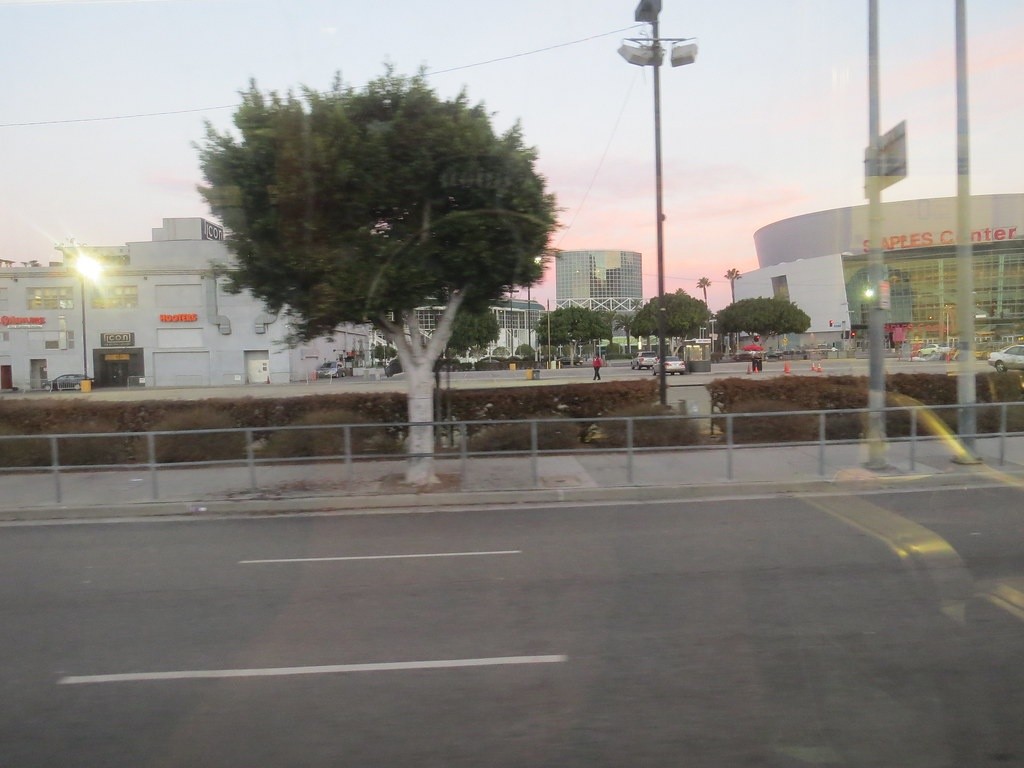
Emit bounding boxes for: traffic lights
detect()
[755,336,759,343]
[851,332,855,339]
[830,319,833,327]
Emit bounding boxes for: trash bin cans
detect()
[80,379,92,393]
[532,370,540,380]
[752,359,762,371]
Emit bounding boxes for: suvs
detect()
[631,351,658,370]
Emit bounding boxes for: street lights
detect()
[527,255,543,347]
[77,259,91,379]
[698,328,707,339]
[709,319,717,353]
[618,0,698,405]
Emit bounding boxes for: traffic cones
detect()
[784,361,790,373]
[747,364,753,374]
[754,365,758,373]
[817,362,822,372]
[811,362,816,371]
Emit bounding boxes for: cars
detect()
[41,374,95,391]
[988,344,1024,373]
[917,343,951,357]
[651,356,686,375]
[555,357,577,365]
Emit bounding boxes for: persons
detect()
[592,353,602,380]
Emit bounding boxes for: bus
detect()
[949,342,994,360]
